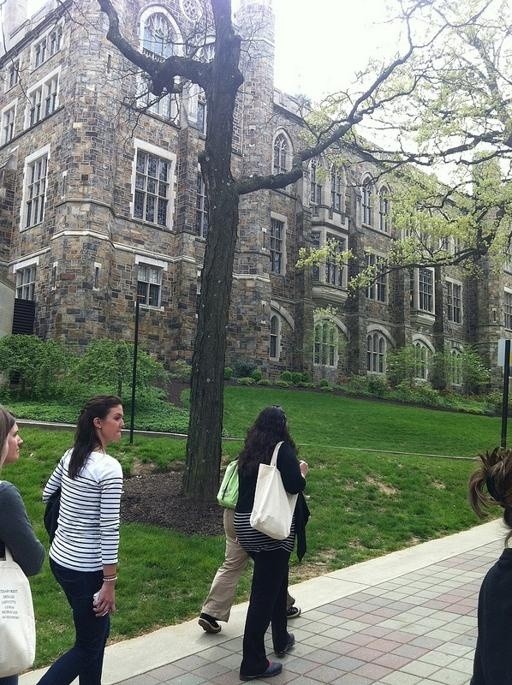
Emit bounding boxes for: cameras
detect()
[93,590,109,617]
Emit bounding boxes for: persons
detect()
[1,405,11,472]
[1,404,49,684]
[196,450,302,634]
[463,447,511,685]
[232,403,309,681]
[35,394,127,685]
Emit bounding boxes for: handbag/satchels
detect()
[0,560,36,678]
[43,486,64,542]
[217,459,241,508]
[250,463,301,542]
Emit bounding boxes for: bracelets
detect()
[100,573,119,584]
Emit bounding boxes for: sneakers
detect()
[286,606,302,619]
[198,612,222,635]
[239,662,283,682]
[276,631,295,659]
